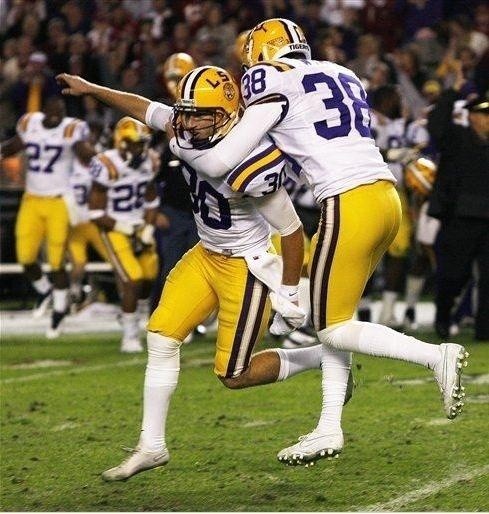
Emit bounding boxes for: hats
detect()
[463,94,488,115]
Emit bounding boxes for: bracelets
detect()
[168,136,186,157]
[280,285,301,305]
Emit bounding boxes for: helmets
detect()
[114,114,151,162]
[161,52,197,81]
[172,64,240,150]
[230,16,312,71]
[402,153,439,197]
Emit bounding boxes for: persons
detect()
[52,66,356,486]
[163,16,469,466]
[1,2,489,349]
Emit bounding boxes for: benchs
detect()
[1,259,118,307]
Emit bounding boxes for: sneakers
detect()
[117,309,145,357]
[33,287,53,314]
[274,428,346,466]
[46,301,70,343]
[433,341,470,420]
[103,444,173,483]
